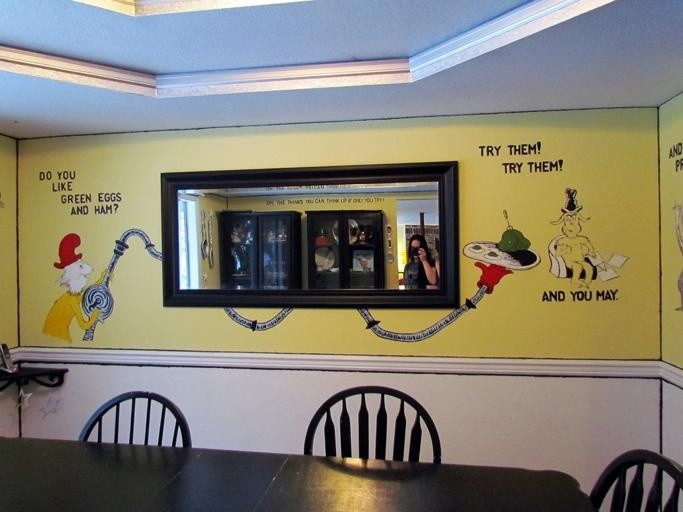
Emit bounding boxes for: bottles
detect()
[359,231,366,245]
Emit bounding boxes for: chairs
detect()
[305,385,441,465]
[77,390,191,450]
[590,450,683,511]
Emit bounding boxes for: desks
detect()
[0,436,589,511]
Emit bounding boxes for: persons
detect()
[404,234,438,290]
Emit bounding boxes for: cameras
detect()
[409,247,419,256]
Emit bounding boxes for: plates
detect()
[314,247,335,270]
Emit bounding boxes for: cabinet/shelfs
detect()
[306,210,384,290]
[220,210,301,290]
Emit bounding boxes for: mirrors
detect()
[161,162,460,309]
